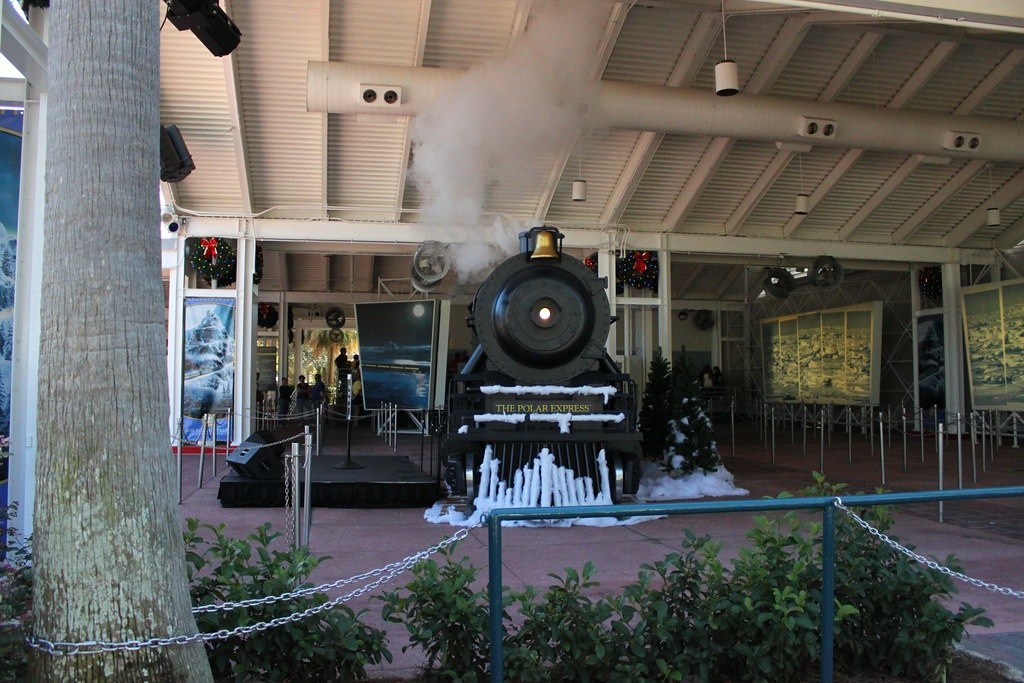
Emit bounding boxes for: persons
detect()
[312,374,325,425]
[699,364,723,387]
[279,378,291,425]
[449,350,470,369]
[297,375,316,427]
[256,383,264,417]
[335,348,348,400]
[351,355,361,380]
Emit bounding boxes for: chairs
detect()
[256,390,276,430]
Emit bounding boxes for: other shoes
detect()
[309,423,317,427]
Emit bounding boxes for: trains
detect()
[442,223,647,511]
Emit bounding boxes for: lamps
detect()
[794,153,810,216]
[159,0,243,59]
[942,130,983,155]
[984,163,1004,228]
[359,82,403,109]
[573,134,586,203]
[798,114,839,141]
[713,0,740,98]
[679,308,695,321]
[160,121,196,184]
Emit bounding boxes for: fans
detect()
[696,310,714,331]
[414,238,453,281]
[810,255,846,293]
[330,329,344,342]
[409,260,442,294]
[761,269,797,302]
[326,307,346,329]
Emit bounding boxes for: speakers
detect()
[225,430,287,480]
